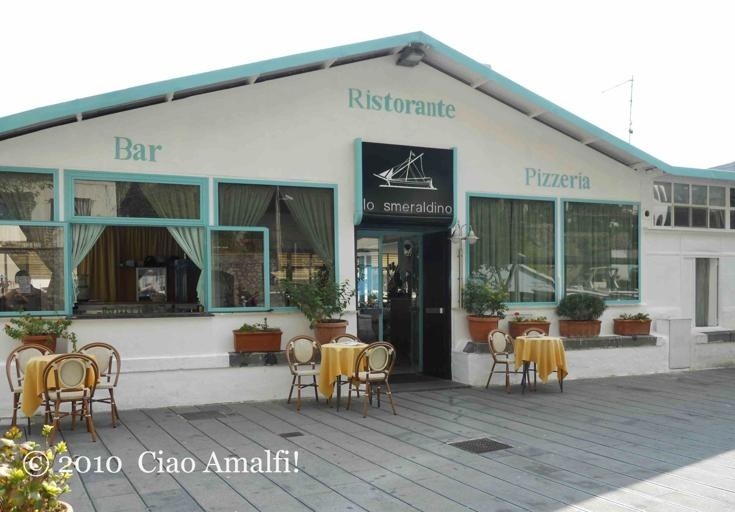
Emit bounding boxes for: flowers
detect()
[457,281,507,343]
[0,424,75,511]
[512,310,547,322]
[1,308,78,354]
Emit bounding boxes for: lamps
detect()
[450,221,480,310]
[396,46,425,67]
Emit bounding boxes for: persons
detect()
[4,271,48,312]
[139,268,160,300]
[610,270,620,291]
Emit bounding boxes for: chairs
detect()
[284,335,334,412]
[76,342,122,430]
[485,329,533,393]
[7,344,57,430]
[331,335,364,398]
[43,353,100,443]
[522,329,547,389]
[348,341,400,418]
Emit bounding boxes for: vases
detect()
[51,501,73,512]
[22,334,56,353]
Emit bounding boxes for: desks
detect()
[20,353,98,431]
[318,342,369,411]
[515,336,569,394]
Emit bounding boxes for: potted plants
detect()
[231,317,282,353]
[557,292,605,337]
[508,320,550,339]
[279,270,355,343]
[613,312,652,336]
